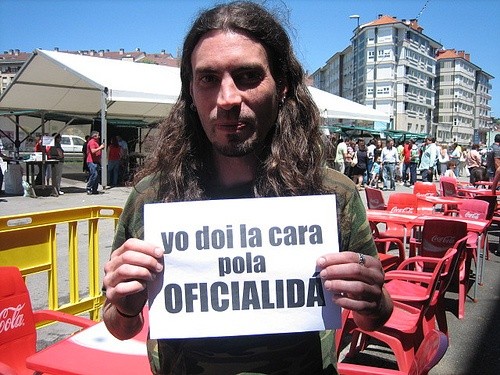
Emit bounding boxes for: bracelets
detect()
[115,306,142,317]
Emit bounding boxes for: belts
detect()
[384,162,393,164]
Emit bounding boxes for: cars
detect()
[49,135,87,152]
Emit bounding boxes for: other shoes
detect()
[380,186,387,191]
[389,187,395,191]
[58,190,64,194]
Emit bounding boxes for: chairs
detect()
[0,262,96,375]
[334,177,500,375]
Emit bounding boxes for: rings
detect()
[359,252,366,265]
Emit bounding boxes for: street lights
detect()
[348,14,360,28]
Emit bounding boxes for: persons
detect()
[101,0,394,375]
[324,131,500,190]
[34,131,131,195]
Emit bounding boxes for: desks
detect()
[367,207,491,233]
[26,306,153,375]
[22,159,60,188]
[419,195,465,207]
[458,187,500,197]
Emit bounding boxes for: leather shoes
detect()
[87,190,92,195]
[92,190,100,194]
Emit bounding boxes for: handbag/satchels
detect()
[350,150,358,167]
